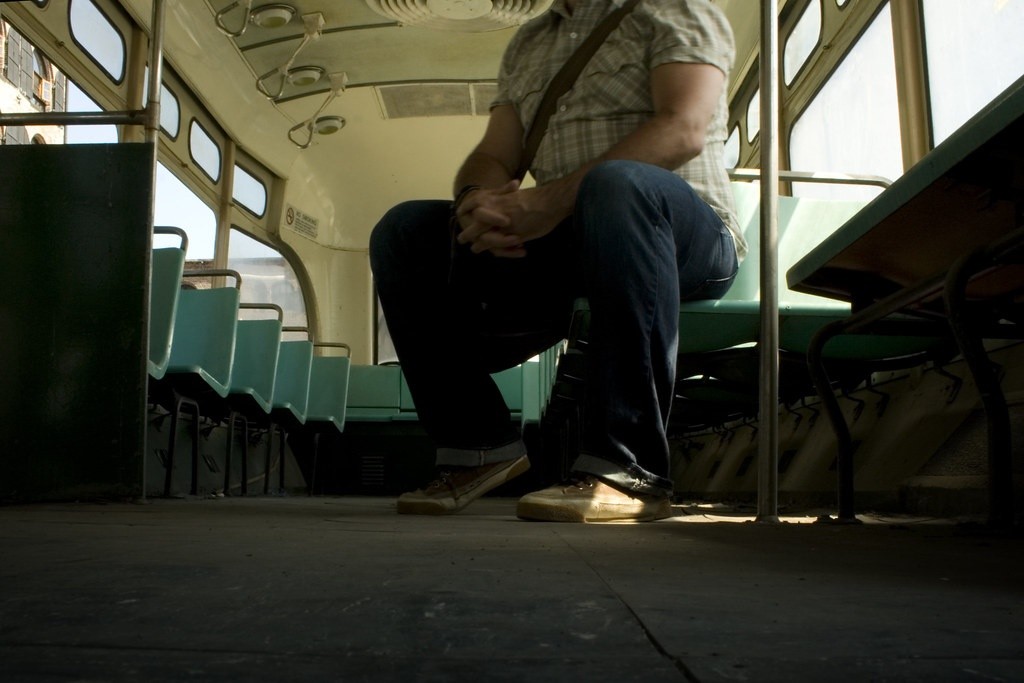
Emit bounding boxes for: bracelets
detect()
[449,184,481,223]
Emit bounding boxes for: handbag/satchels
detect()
[443,214,586,374]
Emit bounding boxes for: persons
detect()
[368,0,748,524]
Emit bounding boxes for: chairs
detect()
[396,371,420,423]
[149,272,242,497]
[786,77,1024,541]
[346,363,402,422]
[290,343,353,496]
[493,364,523,421]
[198,304,283,497]
[239,326,317,497]
[146,228,187,377]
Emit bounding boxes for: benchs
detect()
[518,164,973,524]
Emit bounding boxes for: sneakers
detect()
[517,473,673,523]
[397,453,531,515]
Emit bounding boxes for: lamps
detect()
[307,116,346,136]
[282,66,325,87]
[246,3,297,30]
